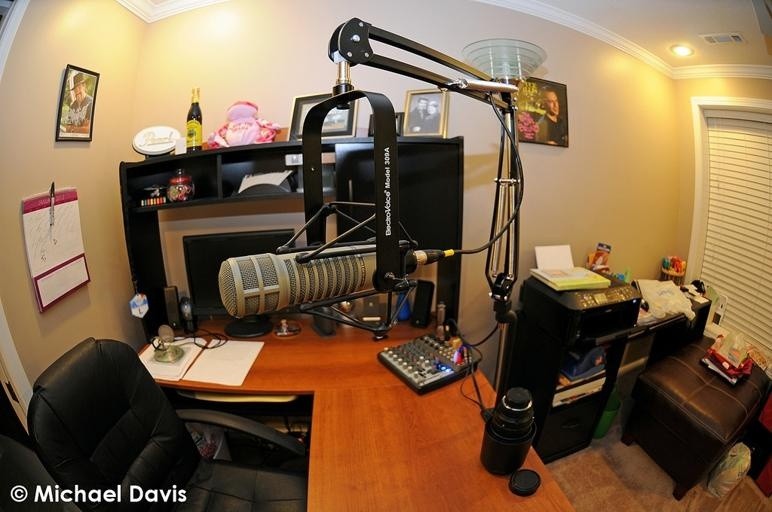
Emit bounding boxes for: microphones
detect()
[216,247,443,321]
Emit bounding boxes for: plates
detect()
[132,125,181,156]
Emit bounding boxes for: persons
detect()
[412,100,442,134]
[532,85,566,146]
[407,97,430,133]
[59,71,93,134]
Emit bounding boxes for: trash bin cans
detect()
[186,423,231,460]
[593,394,622,438]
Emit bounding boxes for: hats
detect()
[66,72,91,91]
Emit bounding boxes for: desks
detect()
[139,316,576,512]
[611,283,712,423]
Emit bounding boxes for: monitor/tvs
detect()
[183,228,295,338]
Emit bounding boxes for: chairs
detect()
[26,336,308,512]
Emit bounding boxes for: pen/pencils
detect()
[50,182,54,226]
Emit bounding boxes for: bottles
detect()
[394,290,411,324]
[479,386,540,477]
[186,87,203,153]
[166,166,198,203]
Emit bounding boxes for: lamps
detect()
[460,38,547,392]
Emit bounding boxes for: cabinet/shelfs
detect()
[119,135,464,341]
[496,265,642,464]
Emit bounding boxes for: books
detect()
[527,266,612,293]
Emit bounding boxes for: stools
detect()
[619,335,772,501]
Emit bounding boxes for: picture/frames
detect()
[367,112,404,137]
[55,64,100,141]
[401,88,449,139]
[285,89,360,141]
[512,75,569,147]
